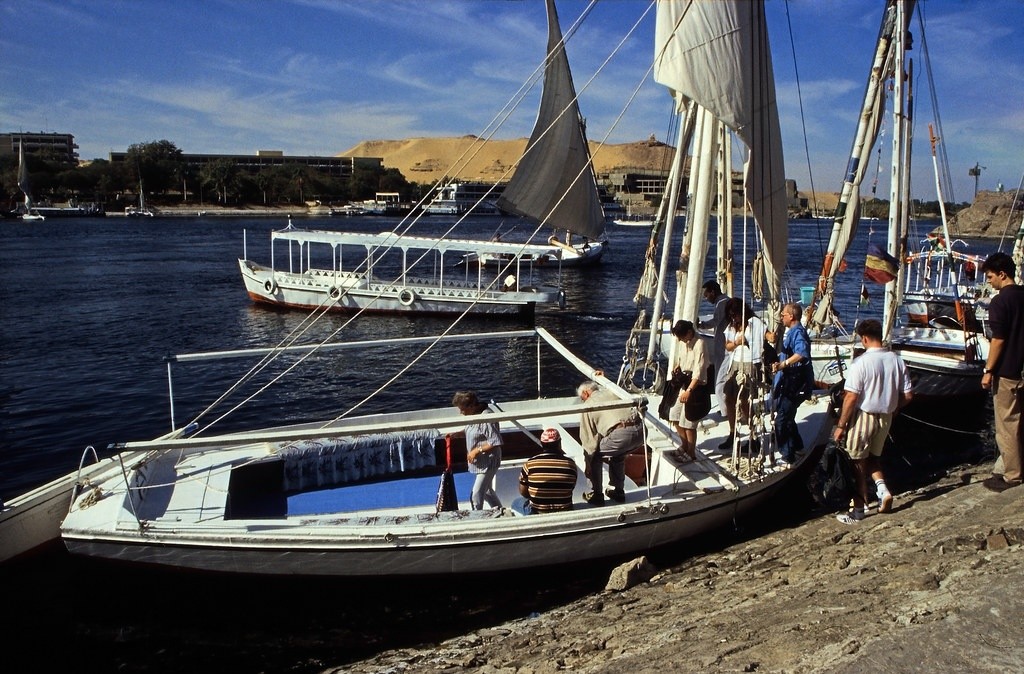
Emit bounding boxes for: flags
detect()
[863,244,900,285]
[859,284,869,306]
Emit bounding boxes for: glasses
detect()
[728,313,740,319]
[781,312,789,317]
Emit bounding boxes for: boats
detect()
[236,213,562,314]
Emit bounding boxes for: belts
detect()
[617,418,642,427]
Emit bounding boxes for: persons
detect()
[452,391,504,510]
[696,279,731,385]
[511,428,578,515]
[834,319,913,525]
[576,371,648,504]
[716,297,778,453]
[669,320,711,463]
[772,303,814,463]
[981,252,1024,490]
[501,271,517,292]
[492,234,501,242]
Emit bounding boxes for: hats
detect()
[541,429,560,442]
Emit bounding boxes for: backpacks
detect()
[817,438,860,500]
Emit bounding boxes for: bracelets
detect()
[837,425,846,429]
[687,387,694,393]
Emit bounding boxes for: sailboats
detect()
[127,163,161,217]
[476,0,610,268]
[56,1,1023,577]
[19,128,46,221]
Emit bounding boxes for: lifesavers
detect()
[583,245,591,256]
[398,288,415,306]
[327,284,343,302]
[829,379,845,416]
[262,277,276,295]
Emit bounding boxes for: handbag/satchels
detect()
[707,363,715,394]
[436,437,458,512]
[782,328,813,393]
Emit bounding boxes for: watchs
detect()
[478,447,483,453]
[983,368,992,374]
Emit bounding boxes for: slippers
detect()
[670,447,697,462]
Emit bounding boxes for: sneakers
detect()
[877,489,893,514]
[836,513,861,525]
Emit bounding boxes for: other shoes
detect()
[605,488,625,503]
[718,436,740,449]
[983,474,1020,492]
[775,458,788,466]
[738,438,760,453]
[795,449,806,460]
[583,492,604,507]
